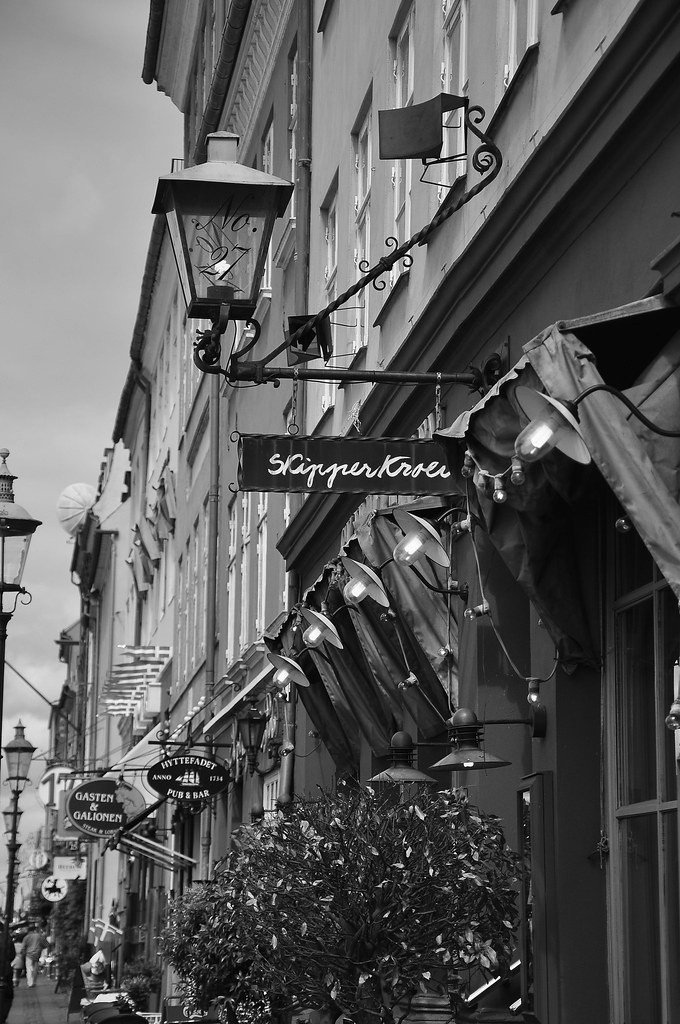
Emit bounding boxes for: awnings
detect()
[100,795,197,874]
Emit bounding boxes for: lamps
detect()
[240,795,323,828]
[237,691,281,776]
[339,551,470,607]
[299,604,360,649]
[139,825,176,843]
[365,732,458,784]
[428,706,546,771]
[267,647,335,688]
[151,130,510,397]
[71,840,97,868]
[512,382,680,462]
[392,506,487,568]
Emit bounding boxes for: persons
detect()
[11,935,26,986]
[20,927,44,988]
[0,921,17,1024]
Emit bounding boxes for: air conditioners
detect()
[132,682,162,735]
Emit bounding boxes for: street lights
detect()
[2,718,38,933]
[0,448,43,774]
